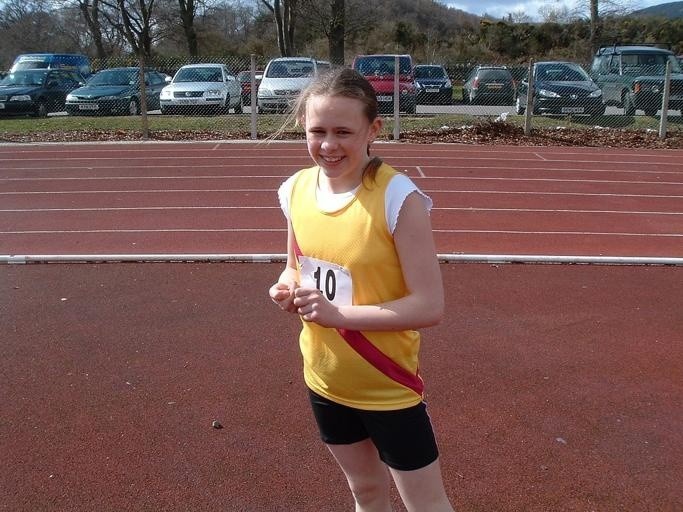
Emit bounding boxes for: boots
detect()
[589,43,683,117]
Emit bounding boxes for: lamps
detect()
[271,65,289,75]
[301,67,310,73]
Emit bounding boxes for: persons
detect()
[266,65,461,512]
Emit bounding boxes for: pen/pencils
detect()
[461,65,517,104]
[351,54,417,115]
[412,65,453,102]
[5,53,93,82]
[256,57,332,113]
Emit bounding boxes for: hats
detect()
[160,64,244,115]
[515,60,607,120]
[237,71,262,94]
[65,67,171,113]
[0,69,89,118]
